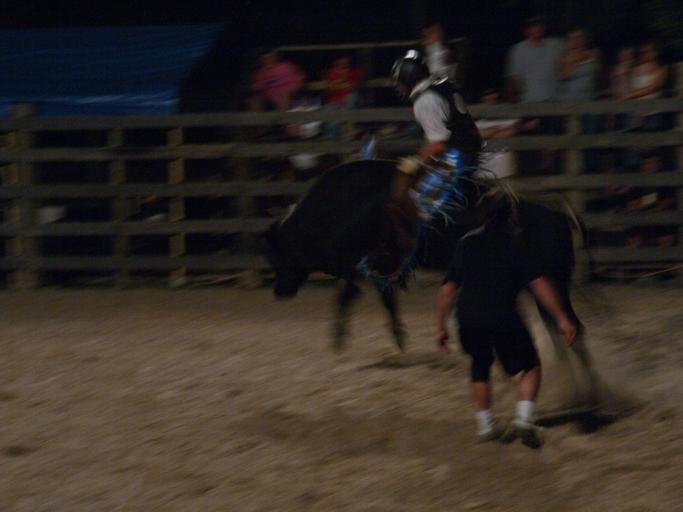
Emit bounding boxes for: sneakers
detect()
[500,422,542,449]
[475,424,499,440]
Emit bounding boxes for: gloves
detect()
[396,153,423,177]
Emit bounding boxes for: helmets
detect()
[391,58,431,90]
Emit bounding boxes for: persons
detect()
[436,188,577,448]
[257,49,427,215]
[473,23,679,248]
[362,27,502,290]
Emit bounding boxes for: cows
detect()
[264,159,603,388]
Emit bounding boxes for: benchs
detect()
[272,0,463,104]
